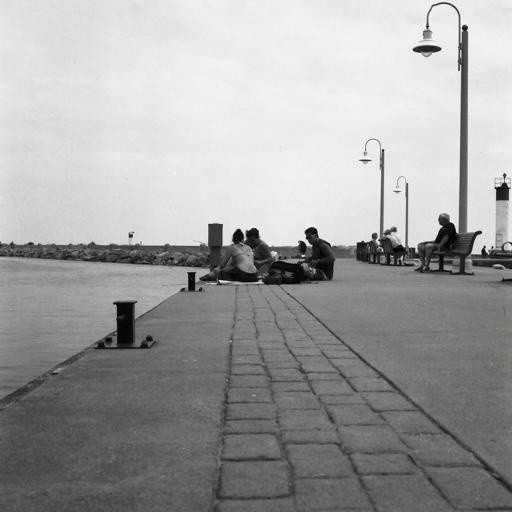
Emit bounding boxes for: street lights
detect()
[360,139,383,253]
[414,3,473,257]
[394,176,414,247]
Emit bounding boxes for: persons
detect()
[370,227,407,265]
[282,227,335,283]
[294,241,307,259]
[243,228,273,277]
[414,213,456,273]
[482,246,487,256]
[199,229,258,282]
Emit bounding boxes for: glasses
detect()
[306,237,311,239]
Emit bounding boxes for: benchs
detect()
[378,238,401,265]
[433,231,483,275]
[368,242,382,263]
